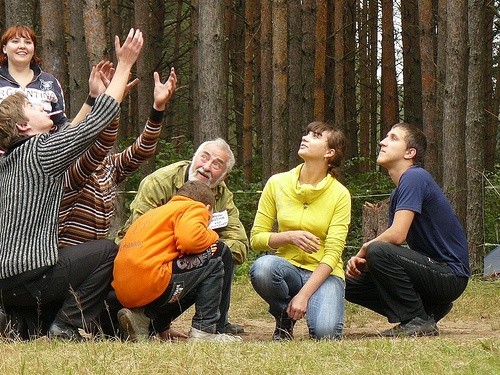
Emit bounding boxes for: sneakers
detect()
[435,303,453,323]
[218,322,243,333]
[383,314,439,337]
[118,306,151,343]
[271,316,297,342]
[190,326,243,344]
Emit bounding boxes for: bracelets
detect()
[85,96,96,106]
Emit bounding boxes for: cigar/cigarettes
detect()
[46,110,62,116]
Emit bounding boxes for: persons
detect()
[250,121,351,342]
[0,25,249,345]
[344,123,471,336]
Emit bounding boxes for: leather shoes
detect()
[50,324,90,342]
